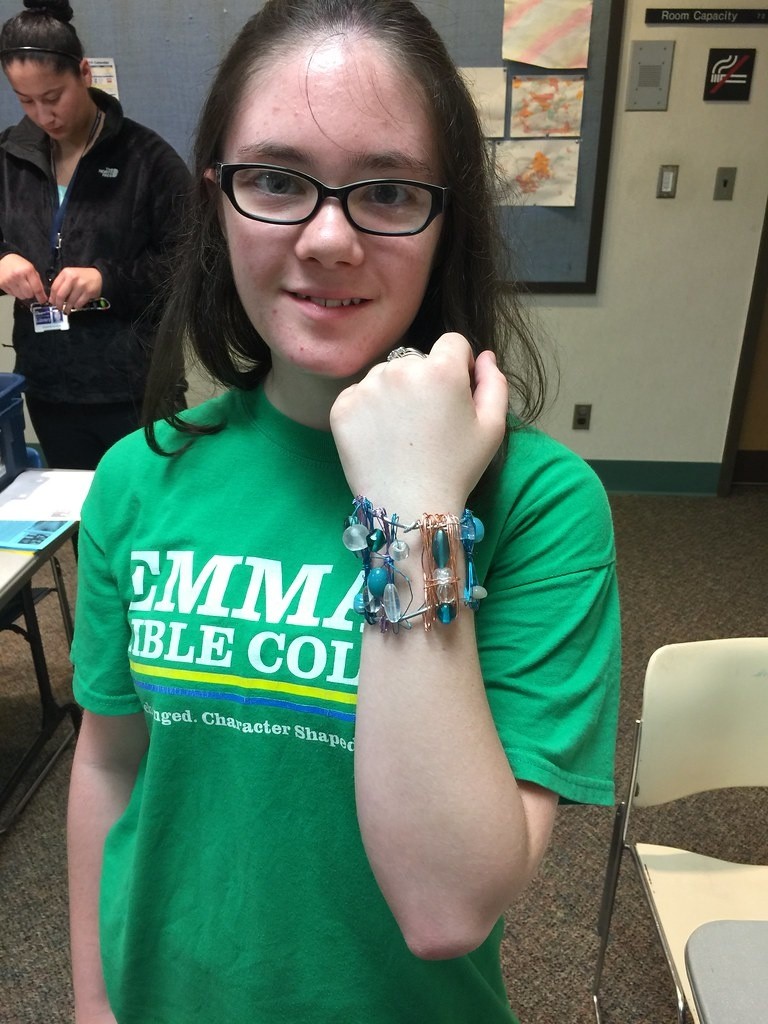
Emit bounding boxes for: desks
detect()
[0,468,98,844]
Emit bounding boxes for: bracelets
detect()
[342,495,489,635]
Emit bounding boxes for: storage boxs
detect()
[0,371,30,494]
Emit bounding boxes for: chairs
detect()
[591,637,768,1024]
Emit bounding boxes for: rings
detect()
[386,347,427,362]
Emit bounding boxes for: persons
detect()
[70,0,624,1024]
[1,0,198,566]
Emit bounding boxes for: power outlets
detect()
[573,404,591,429]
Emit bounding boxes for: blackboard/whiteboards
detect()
[0,1,629,295]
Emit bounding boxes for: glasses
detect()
[210,159,452,239]
